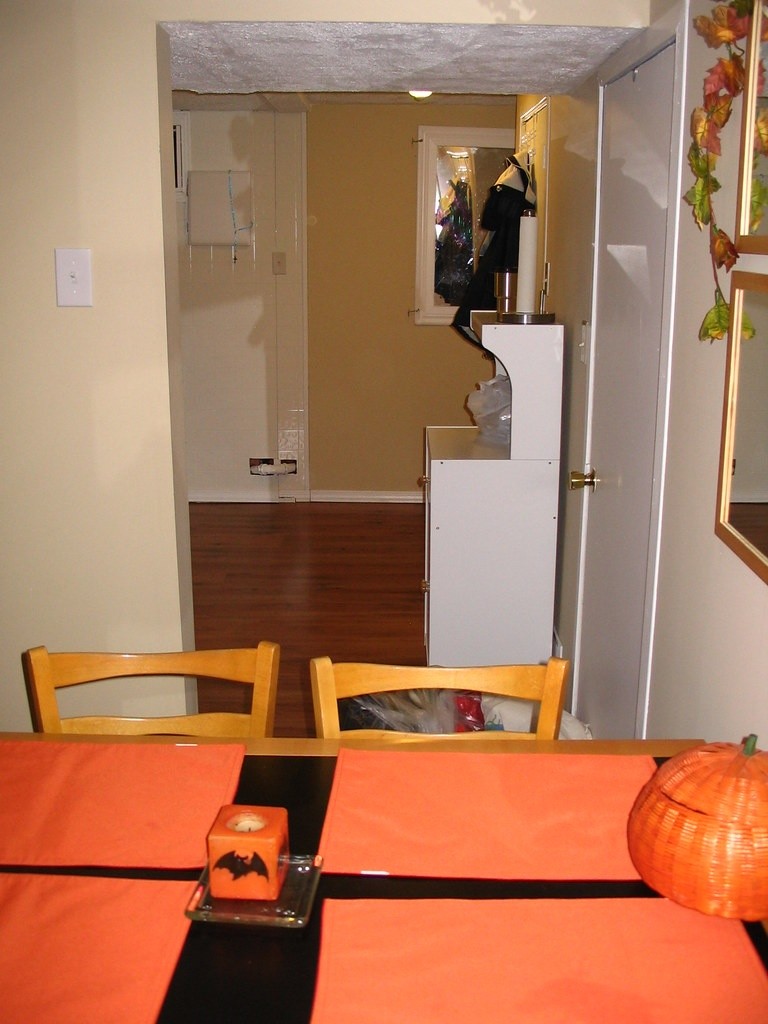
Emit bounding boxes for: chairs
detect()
[309,656,569,740]
[25,641,281,739]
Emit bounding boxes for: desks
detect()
[1,729,768,1024]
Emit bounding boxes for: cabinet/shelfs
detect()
[417,311,565,668]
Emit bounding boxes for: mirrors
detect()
[733,0,768,256]
[415,123,515,326]
[714,270,768,587]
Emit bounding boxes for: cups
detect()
[493,267,517,313]
